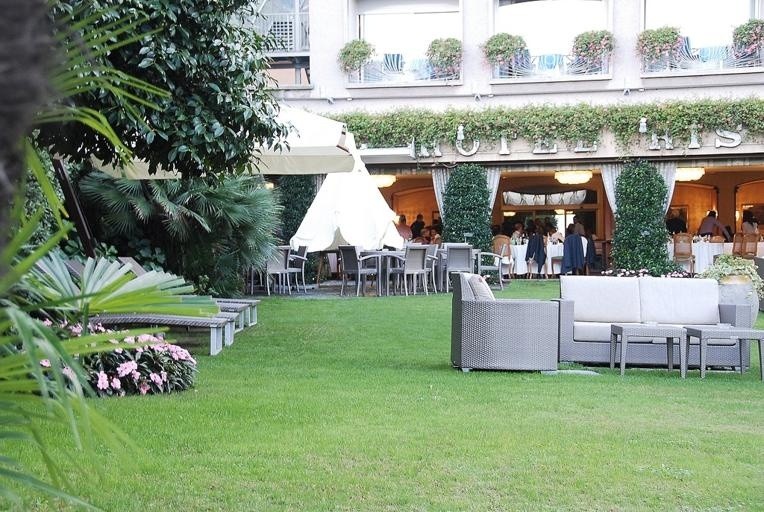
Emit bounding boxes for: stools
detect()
[609,321,688,380]
[682,323,764,380]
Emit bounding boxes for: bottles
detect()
[521,232,525,245]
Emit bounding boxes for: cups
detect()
[517,237,521,245]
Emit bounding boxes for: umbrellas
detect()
[290,132,404,254]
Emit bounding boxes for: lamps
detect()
[367,173,398,189]
[674,166,705,184]
[554,168,595,186]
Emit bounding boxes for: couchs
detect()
[449,272,560,376]
[553,268,753,370]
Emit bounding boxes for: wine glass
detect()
[523,238,526,245]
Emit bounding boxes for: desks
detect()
[491,229,763,278]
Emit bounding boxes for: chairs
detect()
[29,255,260,358]
[237,236,513,298]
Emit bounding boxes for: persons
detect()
[697,211,726,236]
[666,207,687,235]
[397,215,443,244]
[493,215,586,243]
[741,210,759,236]
